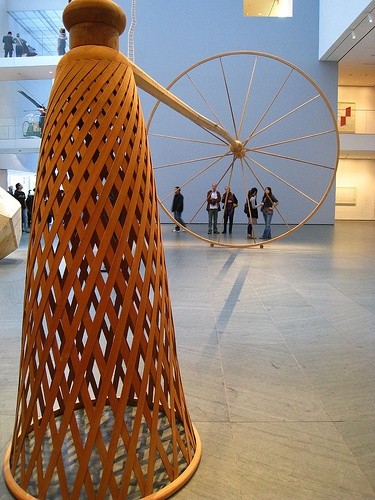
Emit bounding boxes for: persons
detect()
[13,33,25,57]
[6,185,13,195]
[222,185,238,234]
[247,187,264,239]
[205,183,221,234]
[28,44,38,55]
[57,28,67,55]
[3,31,15,57]
[26,188,35,228]
[260,187,279,239]
[172,186,186,232]
[13,183,30,233]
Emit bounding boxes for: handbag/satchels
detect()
[244,203,248,214]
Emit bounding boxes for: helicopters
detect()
[17,89,47,139]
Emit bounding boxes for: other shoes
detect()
[229,232,231,234]
[208,231,212,234]
[268,235,270,238]
[222,231,226,233]
[260,236,267,239]
[182,229,185,231]
[173,230,179,232]
[214,231,220,234]
[247,235,255,239]
